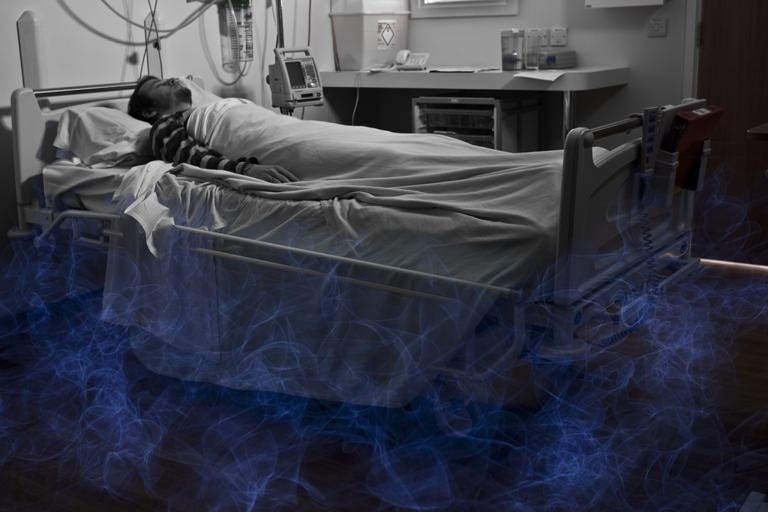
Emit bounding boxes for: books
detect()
[656,103,728,191]
[429,67,499,74]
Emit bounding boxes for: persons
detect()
[127,73,299,185]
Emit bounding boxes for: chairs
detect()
[413,95,503,153]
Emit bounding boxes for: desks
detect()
[320,64,631,150]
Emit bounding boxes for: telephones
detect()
[396,49,429,69]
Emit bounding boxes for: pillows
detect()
[52,74,225,164]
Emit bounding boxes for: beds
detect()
[7,77,707,414]
